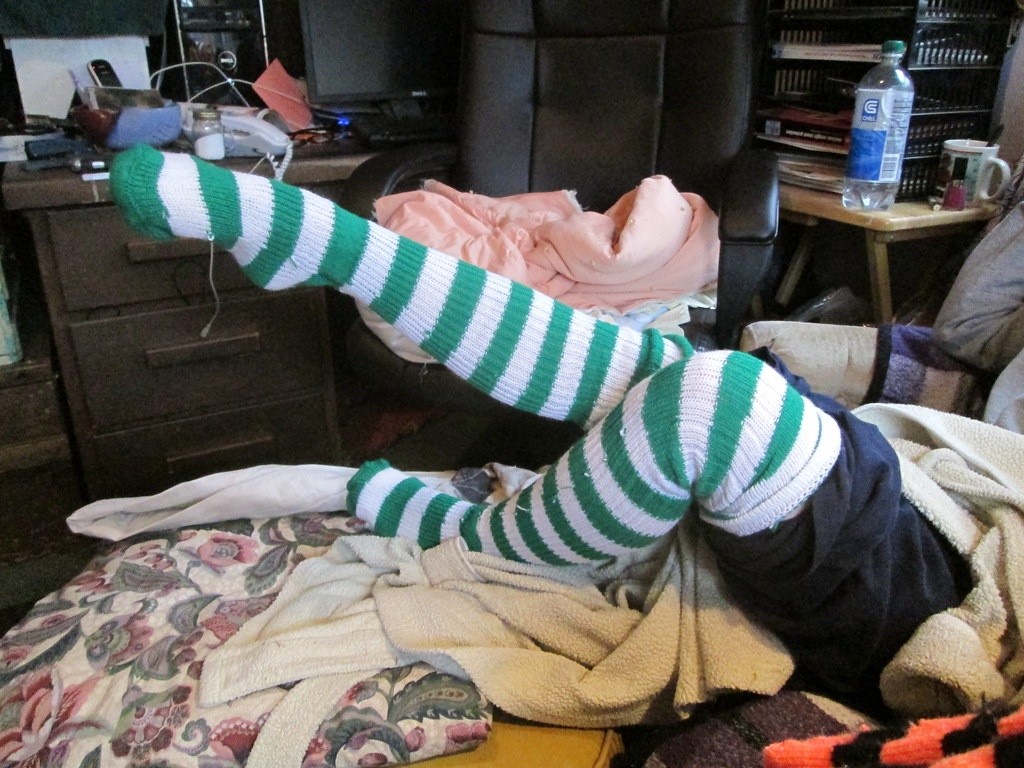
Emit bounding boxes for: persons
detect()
[108,143,1024,717]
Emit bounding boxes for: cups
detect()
[935,139,1011,210]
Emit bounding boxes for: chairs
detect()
[324,0,779,469]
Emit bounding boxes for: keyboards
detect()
[349,117,462,145]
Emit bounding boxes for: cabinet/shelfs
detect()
[757,0,1024,203]
[0,183,385,500]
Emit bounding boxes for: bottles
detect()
[841,40,914,210]
[191,109,225,161]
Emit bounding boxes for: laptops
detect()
[301,0,467,121]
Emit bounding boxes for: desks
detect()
[776,184,1000,326]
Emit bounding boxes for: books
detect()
[773,42,882,63]
[755,97,852,194]
[80,162,110,181]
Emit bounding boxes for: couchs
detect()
[0,321,878,768]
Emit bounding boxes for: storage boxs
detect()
[0,326,72,475]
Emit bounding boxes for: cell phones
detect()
[87,59,123,89]
